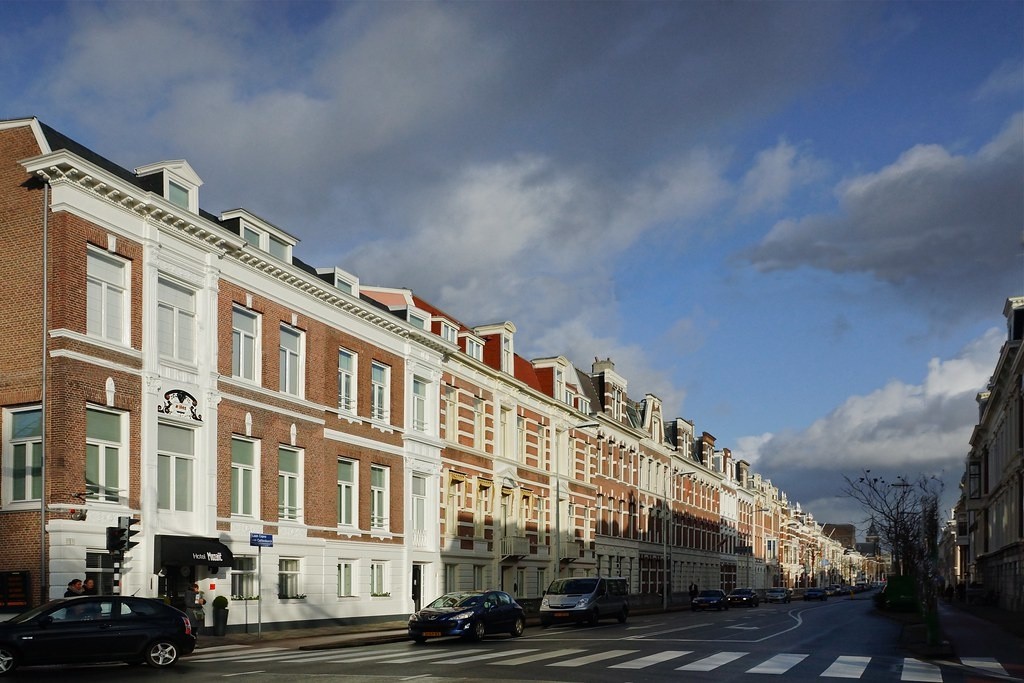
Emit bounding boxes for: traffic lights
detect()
[106,526,127,552]
[128,516,142,552]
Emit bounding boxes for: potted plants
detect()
[212,595,229,637]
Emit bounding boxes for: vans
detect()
[538,575,630,627]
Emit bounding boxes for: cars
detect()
[0,594,196,679]
[727,587,759,609]
[691,588,728,612]
[764,587,791,603]
[803,588,827,601]
[823,580,887,597]
[408,589,526,645]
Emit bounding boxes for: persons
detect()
[957,580,978,603]
[64,580,85,620]
[689,581,700,604]
[946,584,956,606]
[81,578,102,620]
[185,584,207,644]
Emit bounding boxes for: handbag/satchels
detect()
[194,592,203,604]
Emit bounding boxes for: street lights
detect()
[555,421,599,578]
[746,508,770,586]
[662,470,695,608]
